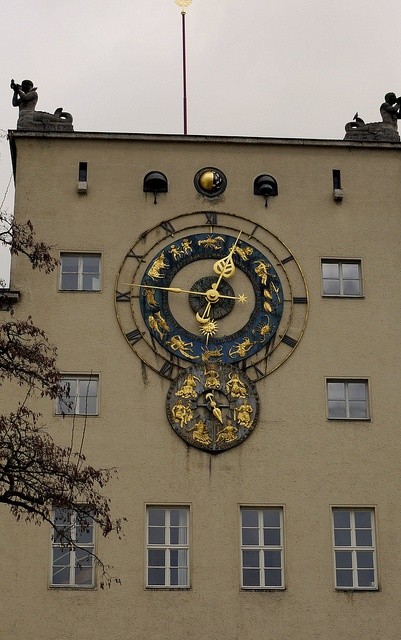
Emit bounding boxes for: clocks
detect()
[114,210,310,455]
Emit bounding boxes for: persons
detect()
[10,78,39,126]
[379,91,401,133]
